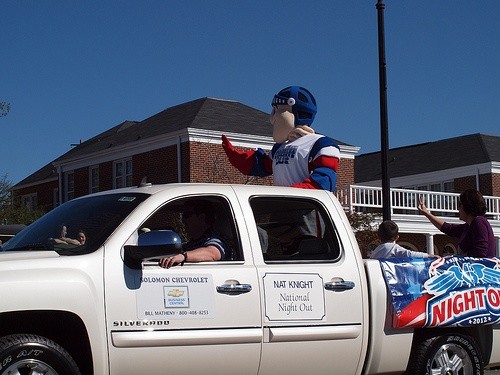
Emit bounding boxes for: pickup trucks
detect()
[0,183,499,374]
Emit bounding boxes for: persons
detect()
[221,86,341,239]
[48,224,81,246]
[417,188,496,258]
[77,229,87,244]
[159,199,232,269]
[369,220,442,258]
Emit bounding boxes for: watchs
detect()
[179,251,187,263]
